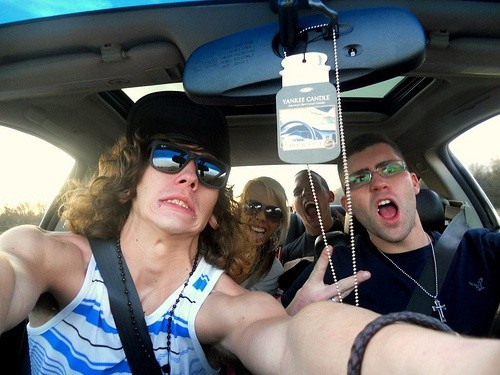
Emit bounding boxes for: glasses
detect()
[344,160,411,193]
[243,199,283,223]
[141,141,229,190]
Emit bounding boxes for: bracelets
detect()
[347,311,460,375]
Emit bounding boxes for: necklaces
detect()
[116,236,200,375]
[377,232,447,322]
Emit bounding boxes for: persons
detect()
[280,131,500,339]
[0,118,500,375]
[280,169,346,303]
[214,175,286,293]
